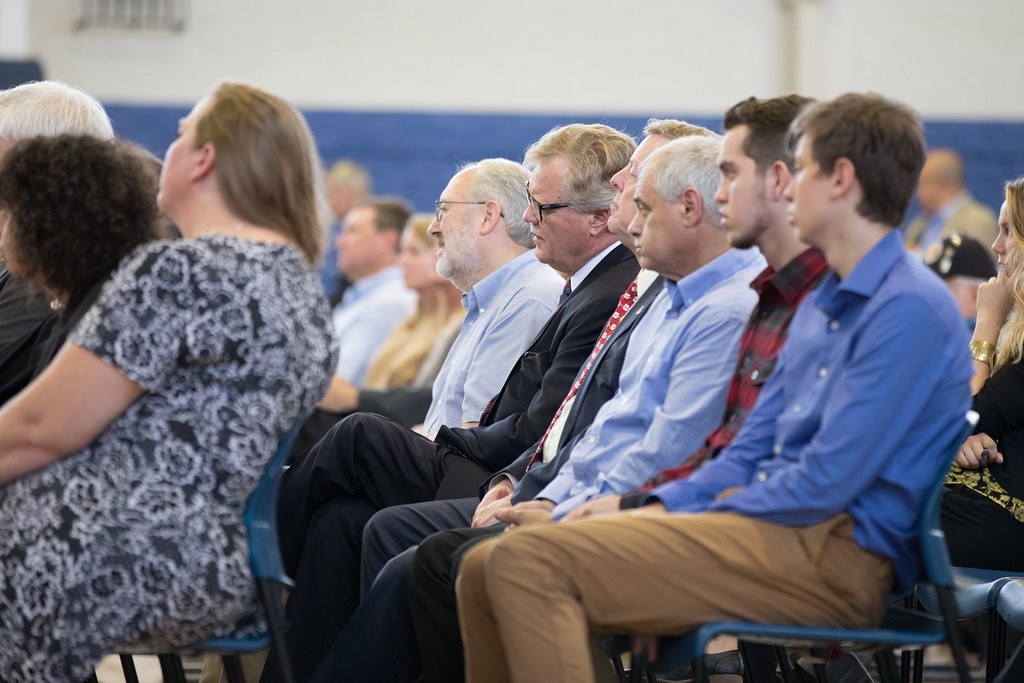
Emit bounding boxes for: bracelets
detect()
[970,339,995,367]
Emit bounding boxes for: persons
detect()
[0,80,341,683]
[193,90,1024,683]
[1,81,173,405]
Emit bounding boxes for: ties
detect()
[526,278,638,472]
[558,277,571,306]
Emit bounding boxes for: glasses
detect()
[435,200,504,221]
[526,182,585,222]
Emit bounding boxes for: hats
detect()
[923,234,998,281]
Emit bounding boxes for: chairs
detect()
[88,414,301,683]
[912,566,1024,683]
[632,411,981,683]
[987,576,1024,683]
